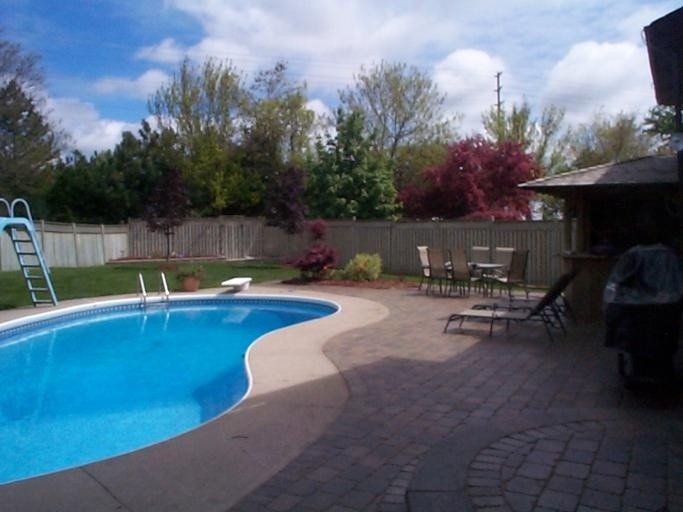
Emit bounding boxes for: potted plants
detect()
[176,260,205,292]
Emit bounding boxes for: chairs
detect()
[416,245,578,342]
